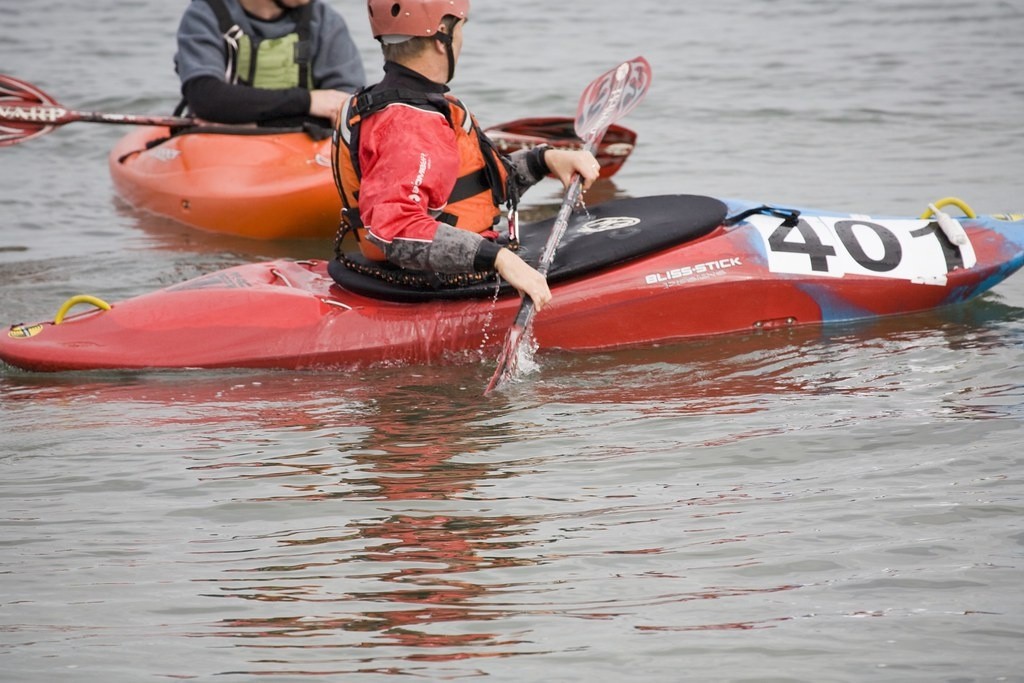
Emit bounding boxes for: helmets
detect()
[367,0,470,40]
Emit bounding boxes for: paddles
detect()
[484,55,652,395]
[0,73,638,181]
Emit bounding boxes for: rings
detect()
[591,163,597,169]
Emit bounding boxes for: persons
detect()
[330,0,602,310]
[173,0,366,130]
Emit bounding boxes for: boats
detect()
[0,194,1023,375]
[107,114,359,245]
